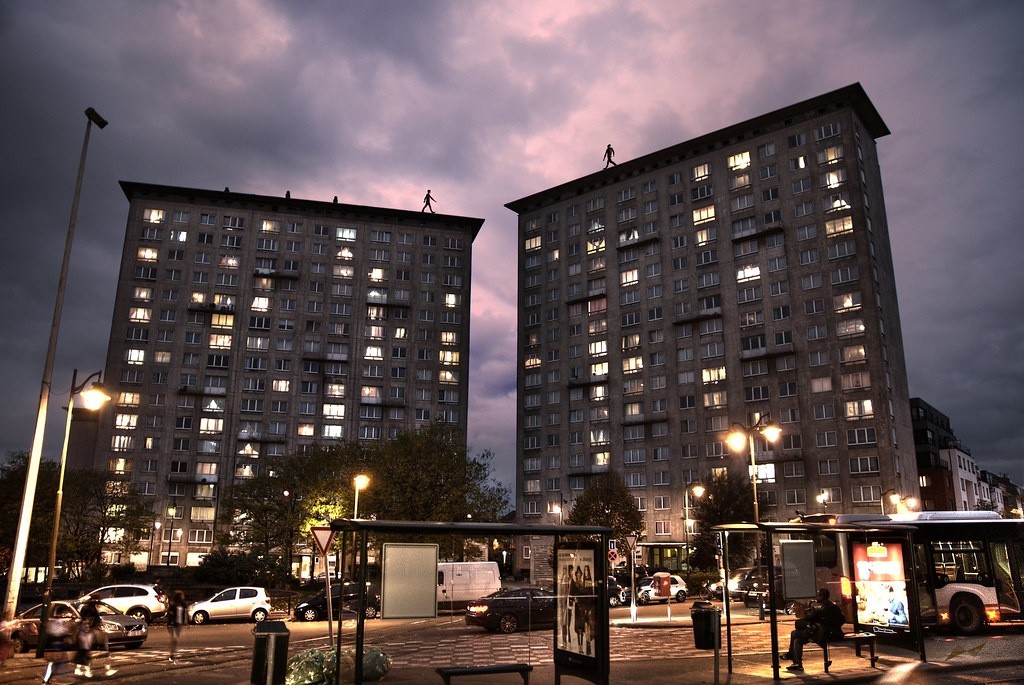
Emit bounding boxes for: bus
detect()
[789,509,1024,637]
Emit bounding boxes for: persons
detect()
[73,594,103,677]
[421,189,437,214]
[568,565,575,649]
[866,584,907,624]
[780,587,845,672]
[166,591,185,666]
[574,564,594,656]
[560,565,571,650]
[602,144,618,170]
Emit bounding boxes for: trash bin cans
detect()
[689,601,722,650]
[251,620,291,685]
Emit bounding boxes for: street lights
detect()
[3,106,108,616]
[727,412,782,620]
[166,496,176,562]
[350,473,369,584]
[685,477,705,574]
[149,513,162,565]
[34,368,107,650]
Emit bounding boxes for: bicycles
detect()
[699,579,713,601]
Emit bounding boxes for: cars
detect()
[318,571,342,581]
[466,586,556,634]
[294,582,381,621]
[607,577,623,606]
[8,599,149,655]
[188,586,272,624]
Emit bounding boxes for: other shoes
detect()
[786,663,804,671]
[780,654,790,660]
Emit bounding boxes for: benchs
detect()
[435,663,534,685]
[820,632,879,672]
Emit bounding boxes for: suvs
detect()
[623,575,690,605]
[78,584,170,626]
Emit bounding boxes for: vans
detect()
[714,567,785,608]
[435,562,504,613]
